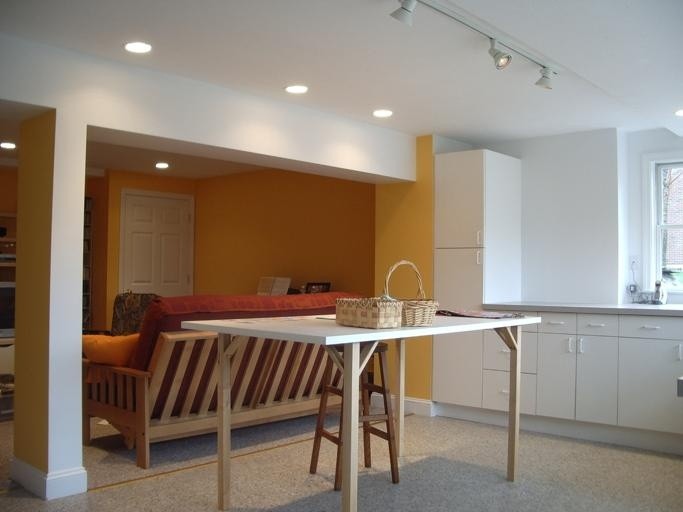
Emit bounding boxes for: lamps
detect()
[386,0,560,94]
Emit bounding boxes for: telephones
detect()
[652,280,668,305]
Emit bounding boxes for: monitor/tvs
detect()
[306,282,330,293]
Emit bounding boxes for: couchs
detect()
[79,290,382,470]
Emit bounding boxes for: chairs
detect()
[108,289,159,337]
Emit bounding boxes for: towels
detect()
[434,306,513,320]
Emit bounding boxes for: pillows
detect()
[81,332,139,367]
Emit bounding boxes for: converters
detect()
[630,285,636,292]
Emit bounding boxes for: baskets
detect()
[384,259,439,328]
[332,297,404,329]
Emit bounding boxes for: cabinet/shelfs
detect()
[616,310,683,458]
[477,304,535,435]
[529,306,621,449]
[430,144,527,423]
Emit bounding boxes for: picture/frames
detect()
[303,281,331,294]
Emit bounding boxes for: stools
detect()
[304,338,399,493]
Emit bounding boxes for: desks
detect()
[178,307,543,511]
